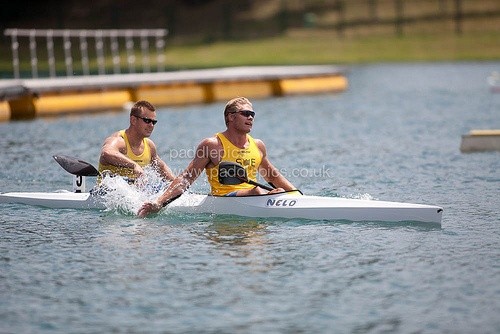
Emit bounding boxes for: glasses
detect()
[232,110,255,118]
[135,116,157,125]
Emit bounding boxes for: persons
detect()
[137,96,299,220]
[98,100,177,191]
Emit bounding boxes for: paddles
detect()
[236,175,304,195]
[52,154,137,183]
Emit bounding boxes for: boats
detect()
[0,190,443,224]
